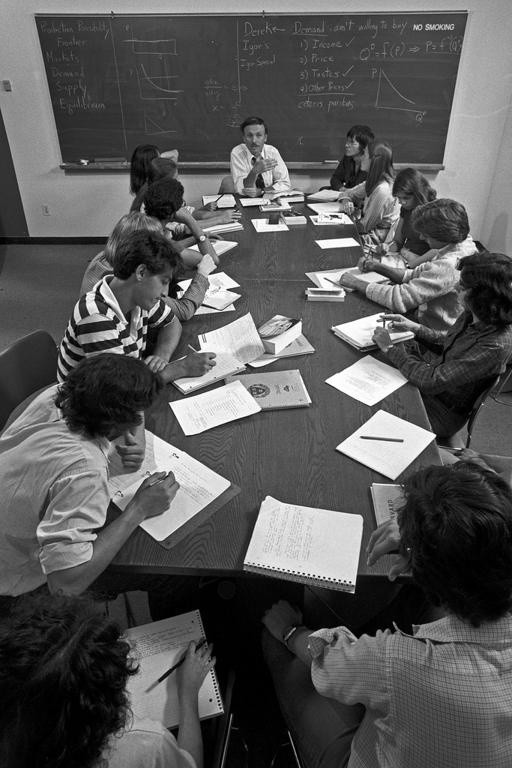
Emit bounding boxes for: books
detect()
[307,188,344,203]
[257,314,303,354]
[109,428,240,550]
[306,286,346,302]
[163,344,246,396]
[113,610,225,734]
[245,494,364,594]
[324,266,389,294]
[331,311,415,354]
[224,369,313,414]
[200,220,244,235]
[239,188,305,213]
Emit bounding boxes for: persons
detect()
[1,352,181,606]
[80,211,217,322]
[230,116,292,198]
[129,145,217,212]
[259,462,512,767]
[0,590,218,768]
[338,140,402,246]
[318,125,375,192]
[340,198,479,335]
[57,228,217,408]
[376,168,437,270]
[129,157,242,235]
[372,252,512,444]
[143,178,220,270]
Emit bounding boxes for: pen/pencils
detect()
[214,193,225,202]
[382,309,387,329]
[361,247,372,274]
[360,436,404,442]
[235,208,240,212]
[149,475,169,486]
[157,633,207,687]
[188,344,199,353]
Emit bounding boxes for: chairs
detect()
[444,366,511,450]
[0,330,59,430]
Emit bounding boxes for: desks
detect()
[101,280,446,588]
[195,190,367,280]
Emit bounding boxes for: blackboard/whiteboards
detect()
[30,10,469,171]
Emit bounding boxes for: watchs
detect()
[196,233,207,246]
[281,623,305,648]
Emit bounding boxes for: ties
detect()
[252,158,266,189]
